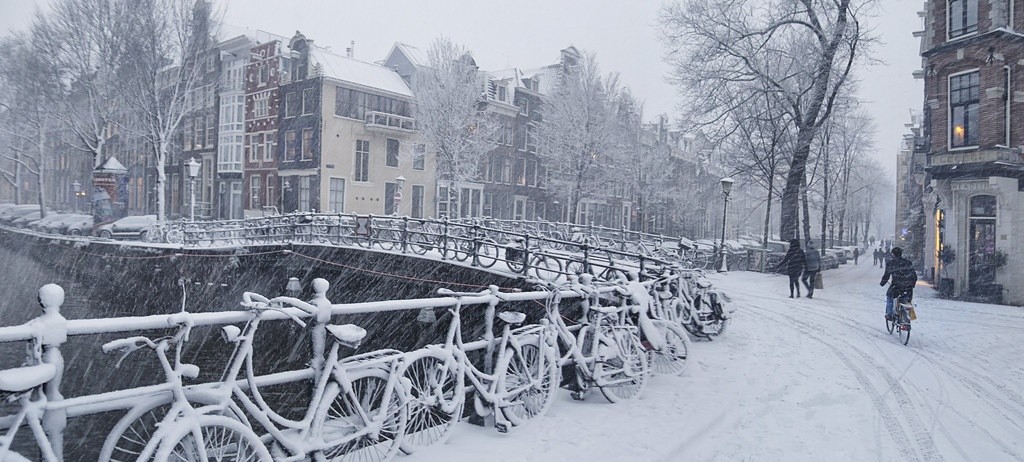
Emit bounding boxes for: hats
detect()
[807,240,815,247]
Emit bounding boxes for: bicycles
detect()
[409,215,458,260]
[454,216,499,269]
[141,205,502,269]
[565,243,630,283]
[95,290,411,462]
[504,224,736,406]
[387,282,559,455]
[738,245,788,275]
[504,233,562,284]
[885,280,913,345]
[0,310,275,462]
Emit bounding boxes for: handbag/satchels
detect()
[814,272,823,289]
[785,262,790,271]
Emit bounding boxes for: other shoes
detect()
[807,294,812,298]
[797,295,799,297]
[885,315,892,321]
[809,287,813,294]
[789,296,793,298]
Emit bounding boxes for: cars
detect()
[844,245,865,260]
[818,249,839,268]
[833,247,848,264]
[97,216,160,243]
[0,203,119,237]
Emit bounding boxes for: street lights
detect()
[394,176,406,216]
[184,156,201,222]
[719,177,735,272]
[71,179,81,215]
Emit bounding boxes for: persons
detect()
[777,239,807,298]
[802,239,822,298]
[868,235,892,268]
[880,247,918,330]
[853,248,859,265]
